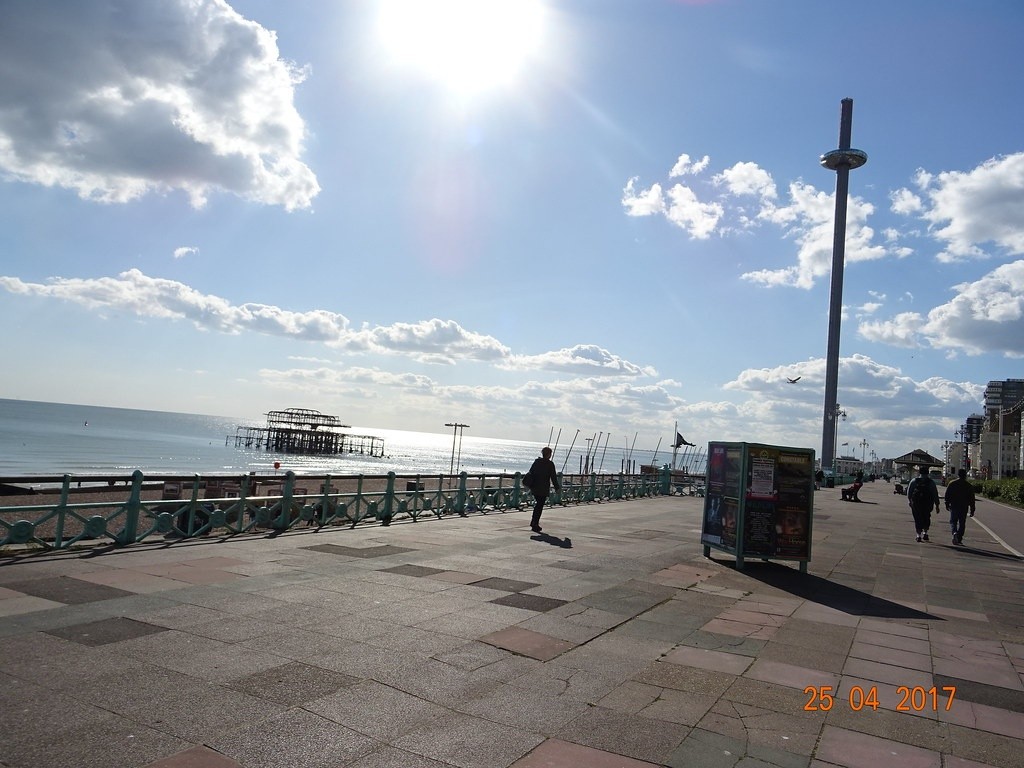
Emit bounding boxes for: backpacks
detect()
[911,479,931,501]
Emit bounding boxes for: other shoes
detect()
[530,522,542,531]
[915,535,921,542]
[957,537,962,543]
[952,532,958,545]
[923,534,930,541]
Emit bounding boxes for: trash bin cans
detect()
[176,502,216,535]
[405,480,425,497]
[825,477,834,488]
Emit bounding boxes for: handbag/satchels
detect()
[523,473,534,486]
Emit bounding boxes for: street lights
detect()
[827,402,847,475]
[954,425,971,470]
[858,438,869,474]
[940,440,954,478]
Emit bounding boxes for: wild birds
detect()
[786,376,801,384]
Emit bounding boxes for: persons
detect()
[907,467,940,543]
[528,447,560,531]
[944,469,975,545]
[842,477,862,501]
